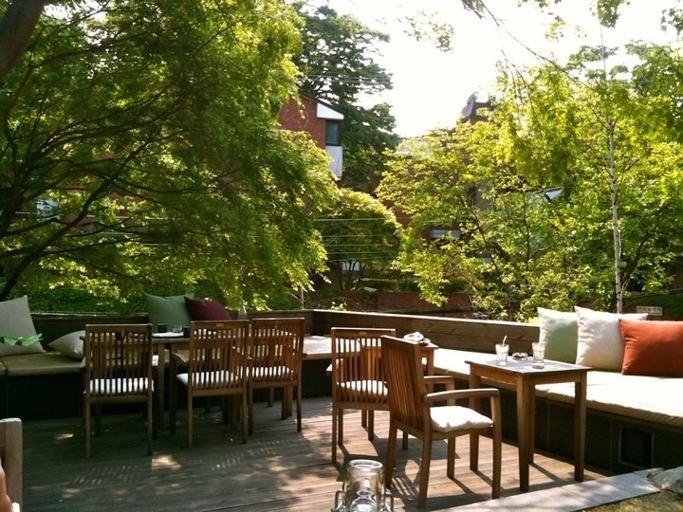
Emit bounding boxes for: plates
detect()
[193,328,216,337]
[151,332,184,338]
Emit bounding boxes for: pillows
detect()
[45,328,127,360]
[617,316,682,376]
[183,295,233,321]
[1,293,47,355]
[138,288,199,334]
[572,304,649,372]
[533,305,577,362]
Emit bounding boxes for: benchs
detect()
[1,306,361,421]
[361,299,682,474]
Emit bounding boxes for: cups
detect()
[155,321,167,334]
[494,344,509,366]
[181,327,191,339]
[530,341,545,369]
[333,459,395,511]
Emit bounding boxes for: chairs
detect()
[166,318,252,450]
[0,416,24,512]
[325,326,411,465]
[378,334,503,511]
[235,316,306,435]
[78,317,157,461]
